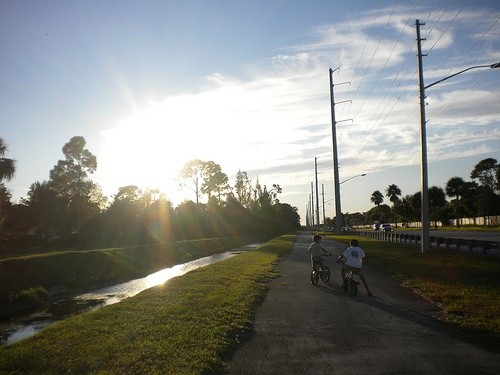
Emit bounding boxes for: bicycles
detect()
[336,258,360,297]
[311,253,332,286]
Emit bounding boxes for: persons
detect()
[336,238,373,296]
[308,234,332,282]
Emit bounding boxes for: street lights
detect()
[305,157,335,231]
[328,65,367,229]
[415,18,500,254]
